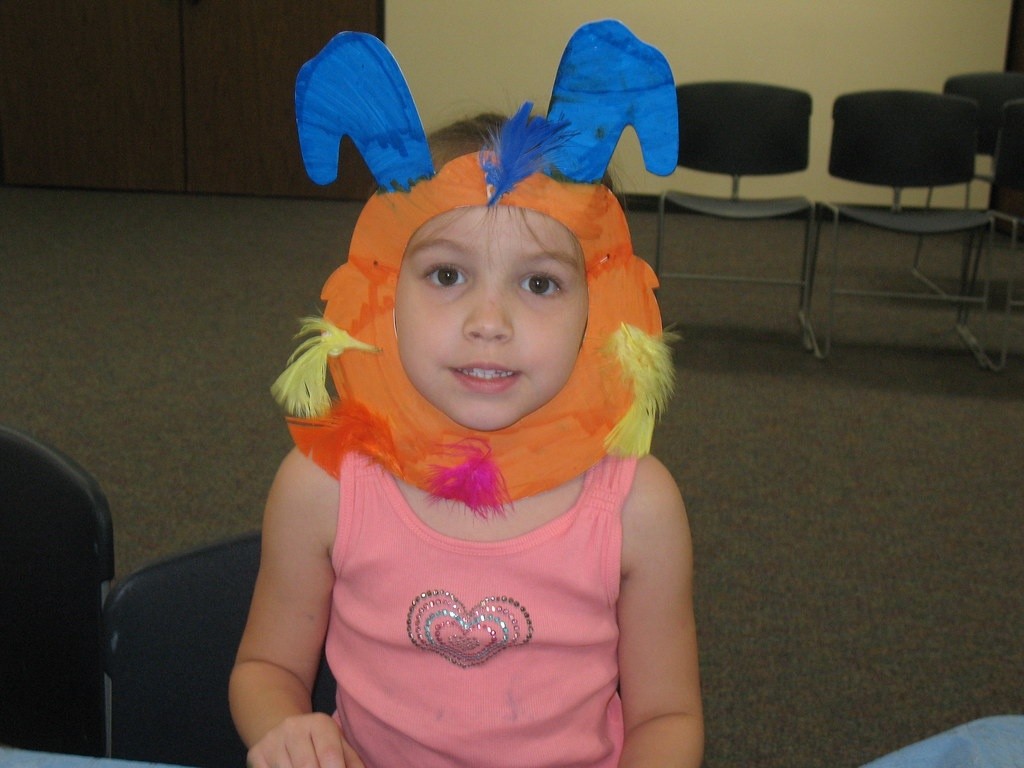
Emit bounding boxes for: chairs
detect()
[0,423,115,757]
[94,529,339,768]
[803,70,1024,372]
[654,81,816,354]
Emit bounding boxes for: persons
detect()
[228,21,704,768]
